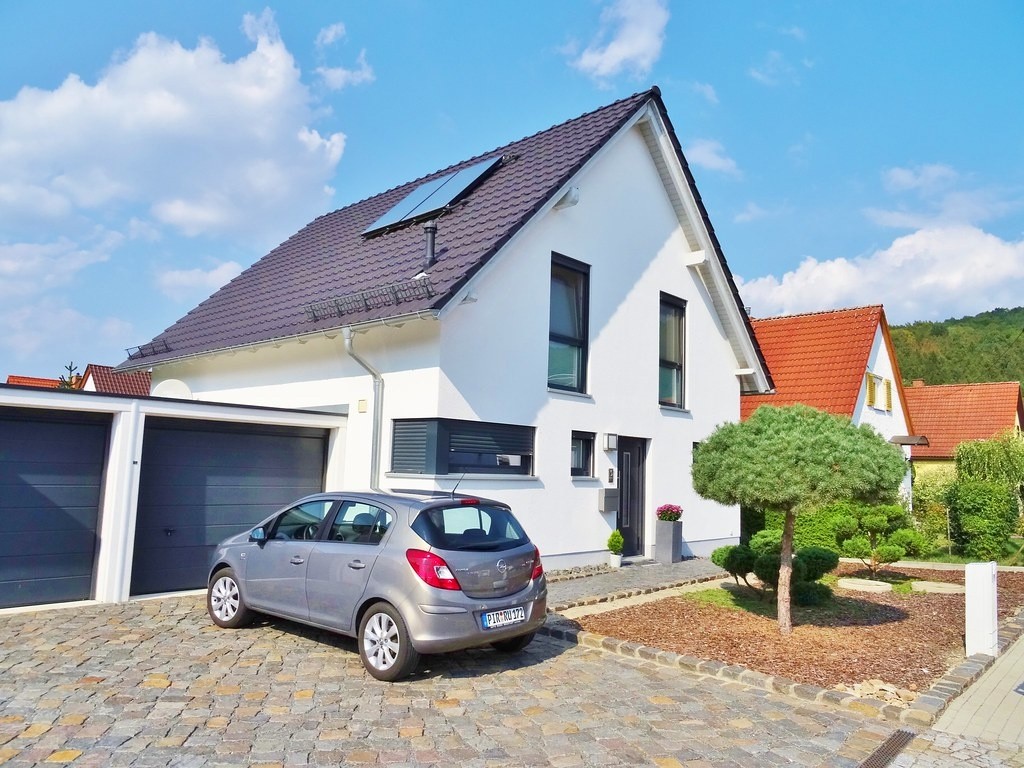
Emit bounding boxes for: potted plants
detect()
[608,529,624,568]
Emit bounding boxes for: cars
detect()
[206,487,548,681]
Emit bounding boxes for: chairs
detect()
[347,513,380,542]
[461,529,488,543]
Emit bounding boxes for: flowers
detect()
[656,504,683,521]
